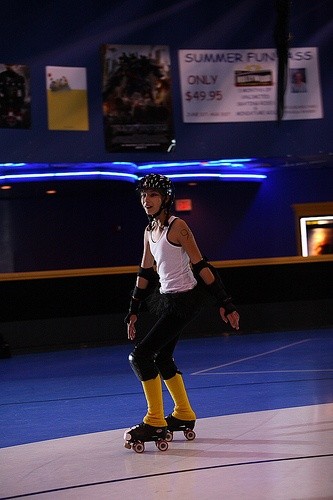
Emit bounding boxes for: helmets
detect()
[135,172,177,207]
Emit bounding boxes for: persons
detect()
[291,71,306,92]
[123,174,240,444]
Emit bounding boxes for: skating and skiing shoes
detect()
[164,413,197,442]
[122,421,169,453]
